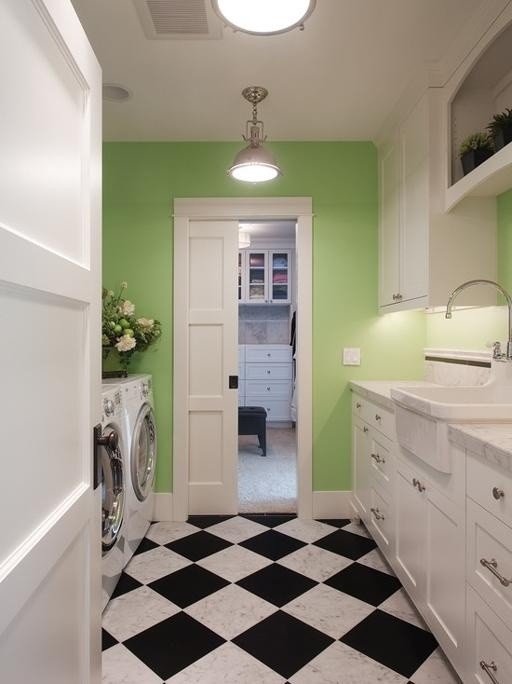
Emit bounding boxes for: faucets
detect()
[445,279,512,360]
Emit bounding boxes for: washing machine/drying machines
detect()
[103,373,157,568]
[101,384,123,616]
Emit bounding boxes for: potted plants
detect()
[457,109,511,178]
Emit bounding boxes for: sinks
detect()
[392,385,512,473]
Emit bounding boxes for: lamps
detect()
[227,86,280,182]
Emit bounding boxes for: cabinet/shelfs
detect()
[237,249,292,306]
[352,390,511,684]
[374,84,497,315]
[239,347,294,430]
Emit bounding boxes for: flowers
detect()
[102,280,162,368]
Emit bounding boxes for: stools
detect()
[238,406,268,458]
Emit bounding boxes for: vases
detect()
[101,345,130,377]
